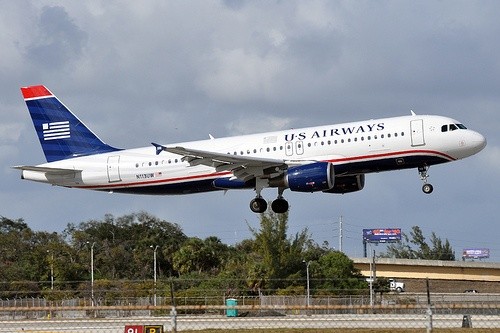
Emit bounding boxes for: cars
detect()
[388,279,405,293]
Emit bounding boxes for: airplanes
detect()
[8,85,488,213]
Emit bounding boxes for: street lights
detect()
[87,241,97,297]
[150,245,161,306]
[463,289,479,293]
[45,250,54,289]
[306,260,312,306]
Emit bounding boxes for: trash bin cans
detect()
[226,298,239,317]
